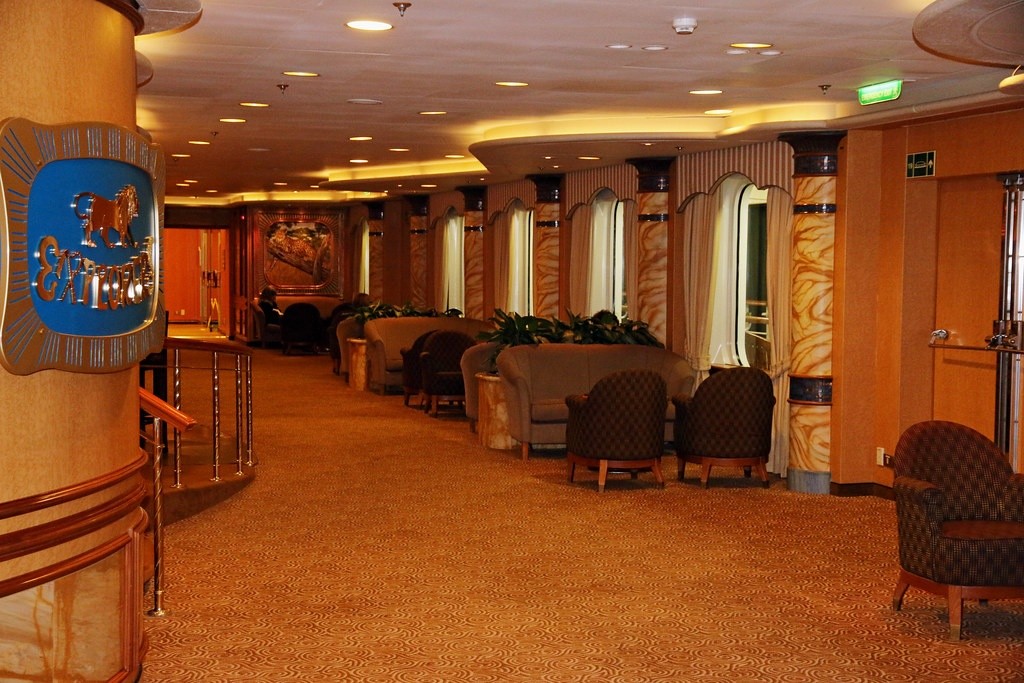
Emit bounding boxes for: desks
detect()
[346,337,368,391]
[475,369,569,449]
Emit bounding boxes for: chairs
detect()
[400,329,441,406]
[418,330,478,416]
[672,364,777,485]
[563,369,667,493]
[279,303,357,374]
[890,418,1024,640]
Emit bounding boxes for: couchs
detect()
[366,316,497,398]
[253,298,283,349]
[335,309,401,373]
[497,341,695,462]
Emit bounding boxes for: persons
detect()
[258,289,283,326]
